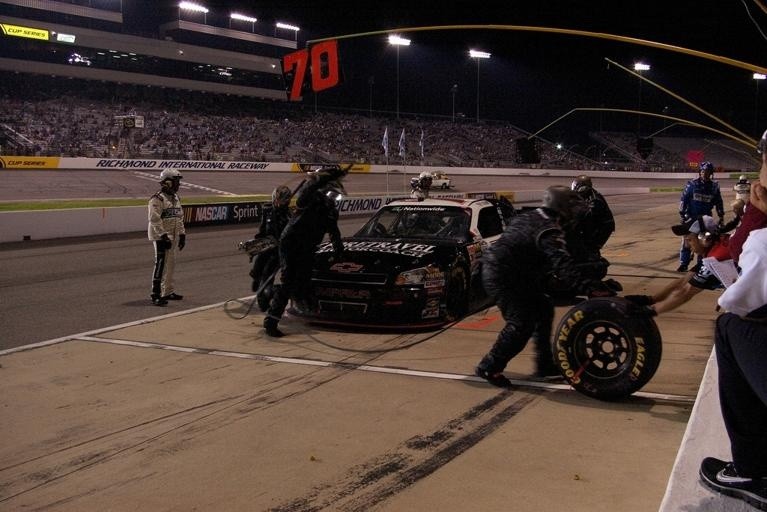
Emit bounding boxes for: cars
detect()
[288,198,537,329]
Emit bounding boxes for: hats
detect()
[671,215,720,237]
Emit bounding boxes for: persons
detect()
[440,172,448,180]
[146,166,188,307]
[472,185,620,388]
[262,163,360,340]
[560,174,618,278]
[624,130,767,512]
[240,185,302,312]
[0,71,766,170]
[409,170,435,200]
[431,172,439,180]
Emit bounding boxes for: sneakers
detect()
[474,364,511,388]
[263,317,283,337]
[677,263,688,271]
[162,293,183,300]
[532,359,559,376]
[252,280,272,313]
[700,457,766,511]
[152,297,168,305]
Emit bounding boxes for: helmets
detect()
[271,185,291,210]
[739,175,748,181]
[542,184,583,221]
[730,199,744,209]
[319,180,347,207]
[571,175,592,194]
[698,161,713,173]
[417,171,432,190]
[158,168,184,183]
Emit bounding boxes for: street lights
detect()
[226,14,258,32]
[176,3,208,23]
[272,22,299,41]
[467,49,491,123]
[752,73,765,139]
[633,62,649,130]
[388,36,409,118]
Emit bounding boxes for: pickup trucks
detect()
[410,171,456,189]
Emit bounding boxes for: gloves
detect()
[162,234,172,249]
[625,294,657,319]
[178,234,185,251]
[591,279,622,292]
